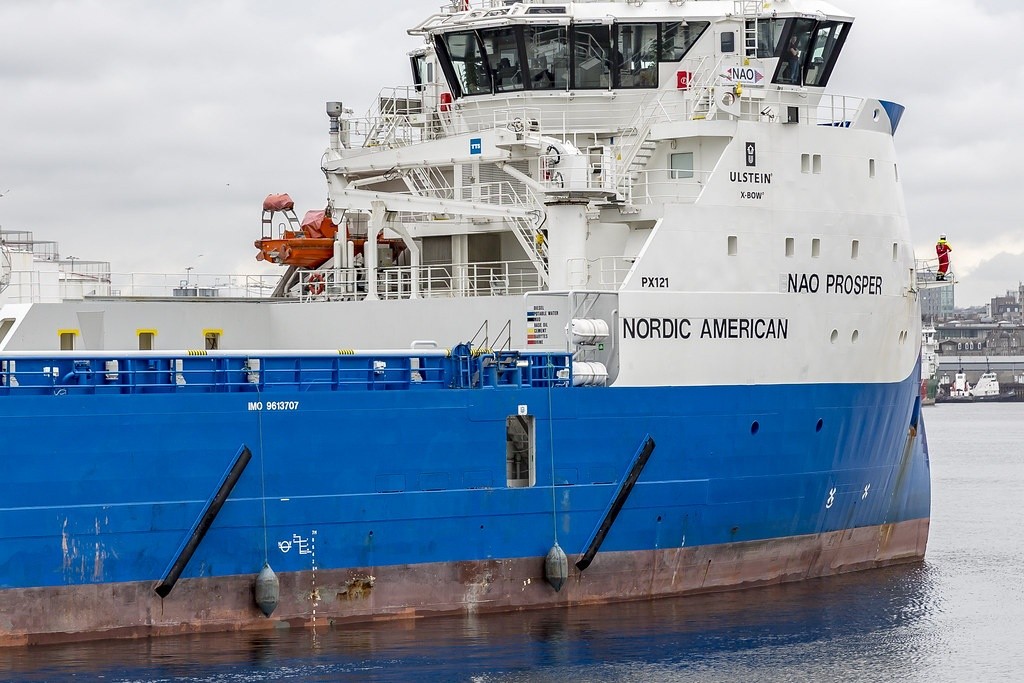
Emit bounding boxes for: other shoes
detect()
[939,276,947,281]
[935,276,940,281]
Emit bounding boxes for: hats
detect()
[500,58,510,63]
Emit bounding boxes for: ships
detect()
[2,1,931,646]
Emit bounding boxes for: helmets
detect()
[940,233,946,238]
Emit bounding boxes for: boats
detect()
[935,355,1023,403]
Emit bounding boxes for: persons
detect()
[936,233,953,281]
